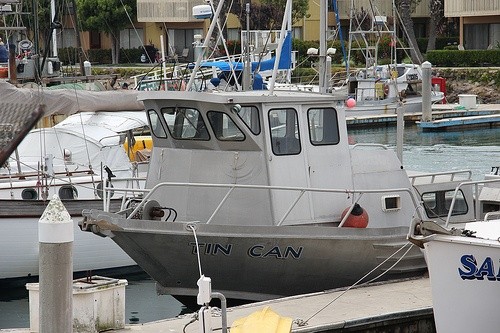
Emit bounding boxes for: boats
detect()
[78,0,500,301]
[407,206,500,333]
[0,0,445,287]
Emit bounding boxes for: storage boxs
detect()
[24,276,128,333]
